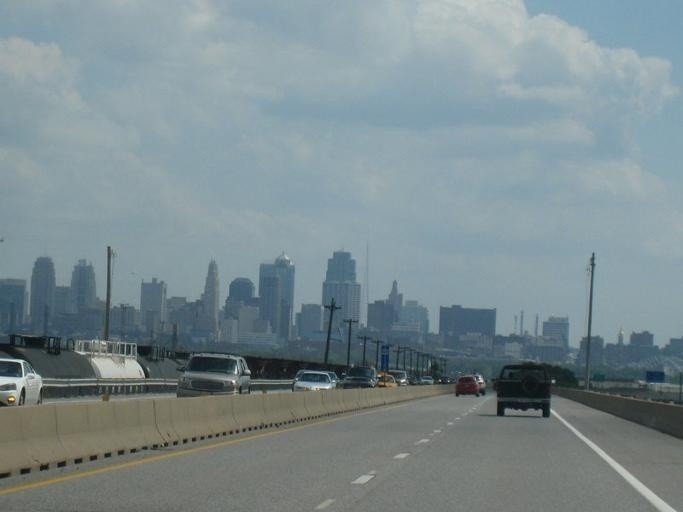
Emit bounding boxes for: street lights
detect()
[585,251,596,390]
[393,344,446,377]
[321,297,343,364]
[358,335,370,365]
[372,340,384,369]
[342,319,360,366]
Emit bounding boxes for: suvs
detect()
[173,353,252,399]
[493,361,556,417]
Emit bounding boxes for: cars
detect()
[292,364,488,398]
[0,358,43,408]
